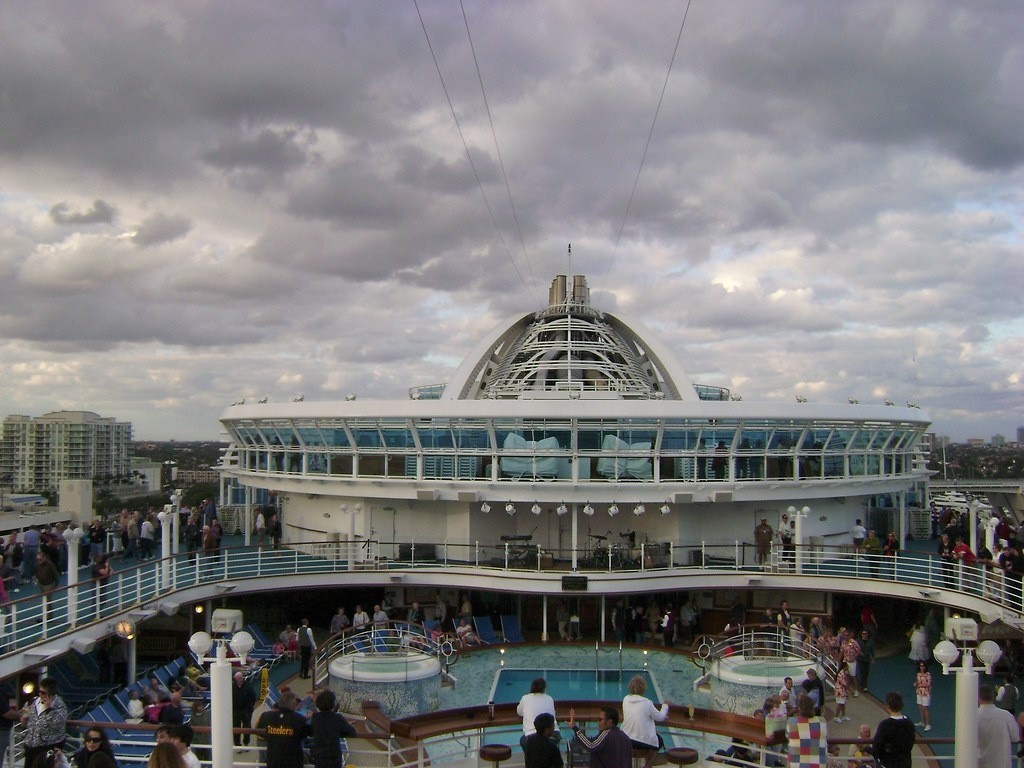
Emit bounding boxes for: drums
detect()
[614,546,624,553]
[597,547,607,557]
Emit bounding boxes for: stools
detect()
[630,745,652,768]
[665,747,699,768]
[477,743,516,768]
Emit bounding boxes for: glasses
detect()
[598,717,607,721]
[862,633,867,635]
[85,736,102,743]
[39,691,49,694]
[785,517,788,519]
[920,665,924,667]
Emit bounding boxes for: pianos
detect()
[496,544,537,569]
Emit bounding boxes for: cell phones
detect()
[41,692,55,704]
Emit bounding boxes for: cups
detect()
[488,701,494,720]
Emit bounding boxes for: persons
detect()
[697,438,823,482]
[517,675,670,768]
[785,694,827,768]
[753,514,795,573]
[976,685,1024,768]
[256,499,282,560]
[928,501,1024,611]
[556,596,702,648]
[330,595,481,653]
[873,692,915,768]
[244,434,327,475]
[908,608,1020,716]
[0,497,223,655]
[0,619,359,768]
[912,660,932,731]
[849,518,900,580]
[705,596,878,768]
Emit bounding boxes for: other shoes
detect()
[150,556,155,560]
[841,718,849,720]
[853,690,858,696]
[143,558,149,561]
[914,722,925,727]
[834,717,842,723]
[303,675,310,679]
[924,725,931,731]
[237,749,242,753]
[245,749,250,752]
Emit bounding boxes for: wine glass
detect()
[689,705,694,720]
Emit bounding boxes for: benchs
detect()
[44,614,526,768]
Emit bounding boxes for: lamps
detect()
[477,499,680,517]
[230,383,927,410]
